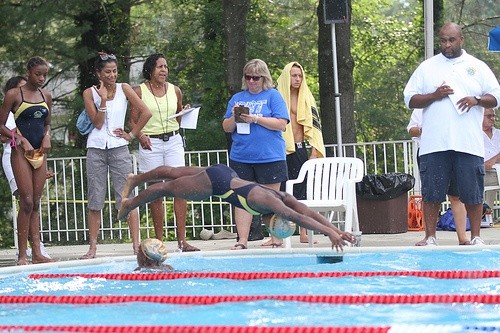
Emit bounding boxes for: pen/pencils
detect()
[233,101,239,107]
[433,84,441,88]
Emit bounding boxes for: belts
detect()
[146,130,179,138]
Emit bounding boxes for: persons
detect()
[80,53,153,259]
[260,62,326,247]
[222,58,290,248]
[134,238,172,271]
[407,108,470,246]
[127,54,200,252]
[1,77,51,260]
[482,108,500,186]
[1,56,58,265]
[117,163,354,253]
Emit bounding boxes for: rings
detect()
[465,102,468,106]
[119,133,123,135]
[143,146,145,149]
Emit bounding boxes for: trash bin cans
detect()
[355,172,409,235]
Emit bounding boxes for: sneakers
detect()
[471,236,485,245]
[425,237,438,246]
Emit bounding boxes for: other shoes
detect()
[15,246,53,263]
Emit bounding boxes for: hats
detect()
[24,148,45,169]
[269,214,296,239]
[141,239,167,262]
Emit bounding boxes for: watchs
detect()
[474,95,482,105]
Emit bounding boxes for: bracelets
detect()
[44,130,51,136]
[128,131,137,140]
[254,114,259,124]
[138,133,145,141]
[97,107,108,113]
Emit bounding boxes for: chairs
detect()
[286,157,365,248]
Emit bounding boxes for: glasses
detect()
[245,75,263,81]
[485,114,495,118]
[97,54,117,63]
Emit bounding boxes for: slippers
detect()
[178,245,200,251]
[415,240,436,245]
[257,242,283,248]
[80,253,95,259]
[459,241,472,245]
[232,244,247,249]
